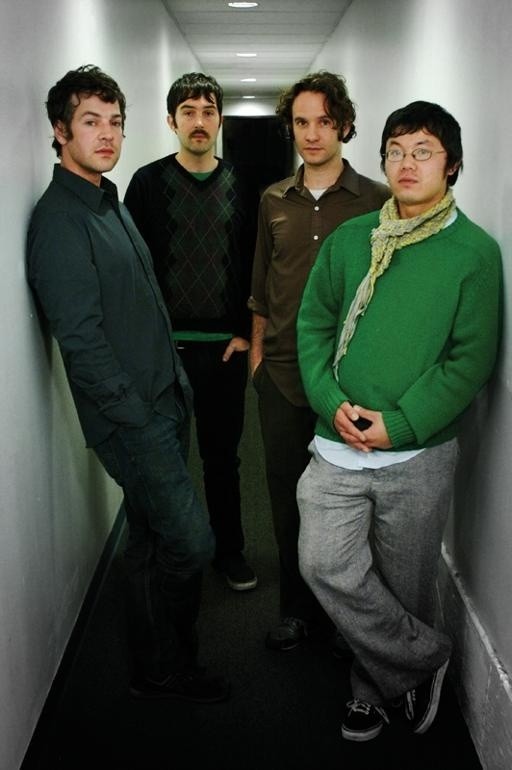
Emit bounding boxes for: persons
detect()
[24,66,229,703]
[123,71,262,593]
[245,70,391,650]
[296,100,502,743]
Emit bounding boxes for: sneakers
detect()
[265,617,309,651]
[342,697,391,742]
[403,658,450,735]
[130,659,224,703]
[215,549,258,589]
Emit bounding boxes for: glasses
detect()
[384,146,446,161]
